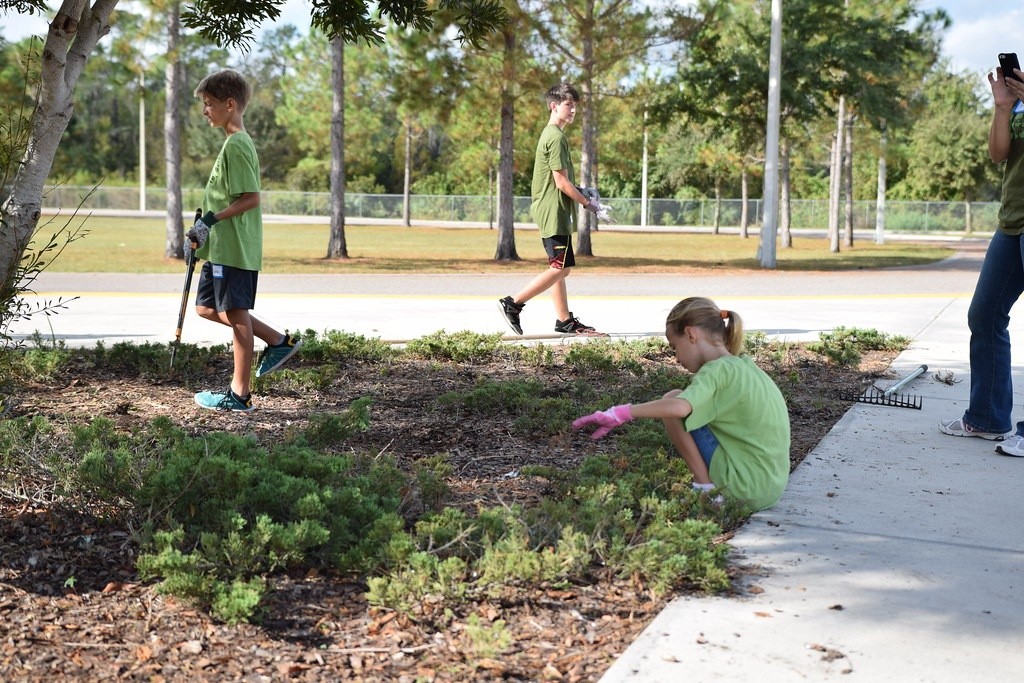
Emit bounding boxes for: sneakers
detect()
[194,385,255,413]
[497,296,526,336]
[938,417,1013,441]
[255,334,301,378]
[994,434,1024,456]
[555,312,595,333]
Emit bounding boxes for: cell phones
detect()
[998,52,1023,88]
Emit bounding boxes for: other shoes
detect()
[678,482,723,502]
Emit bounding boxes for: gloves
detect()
[182,226,200,265]
[572,403,634,440]
[184,211,219,248]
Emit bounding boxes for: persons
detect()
[183,69,301,413]
[570,297,791,511]
[938,67,1024,457]
[497,84,612,335]
